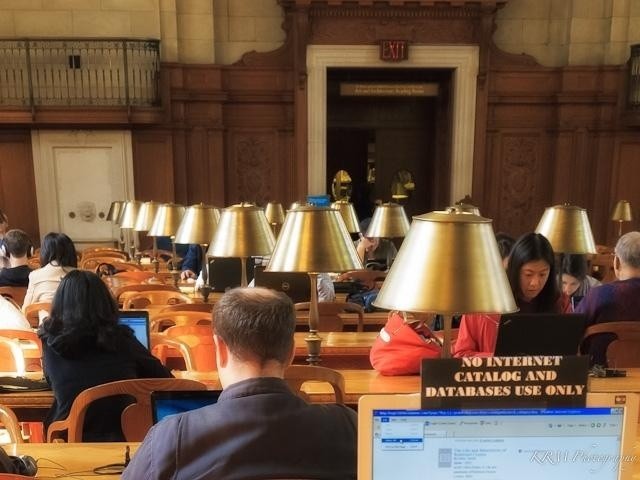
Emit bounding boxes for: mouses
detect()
[16,454,37,476]
[187,277,194,284]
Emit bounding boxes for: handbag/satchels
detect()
[370,311,443,376]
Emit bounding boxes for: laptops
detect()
[117,309,150,357]
[254,265,318,311]
[150,390,223,428]
[571,296,585,312]
[357,394,640,480]
[367,258,395,282]
[494,313,588,356]
[208,256,266,292]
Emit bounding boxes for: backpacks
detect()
[344,289,378,313]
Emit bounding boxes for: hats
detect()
[358,218,373,236]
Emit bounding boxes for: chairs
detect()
[0,245,638,478]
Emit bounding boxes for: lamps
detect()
[104,193,635,369]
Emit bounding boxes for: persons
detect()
[573,231,639,368]
[497,235,517,267]
[557,254,602,309]
[454,233,573,359]
[1,228,34,287]
[180,218,398,302]
[37,268,175,442]
[22,232,79,317]
[120,286,357,480]
[0,211,11,268]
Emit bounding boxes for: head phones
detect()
[1,229,35,259]
[96,262,117,280]
